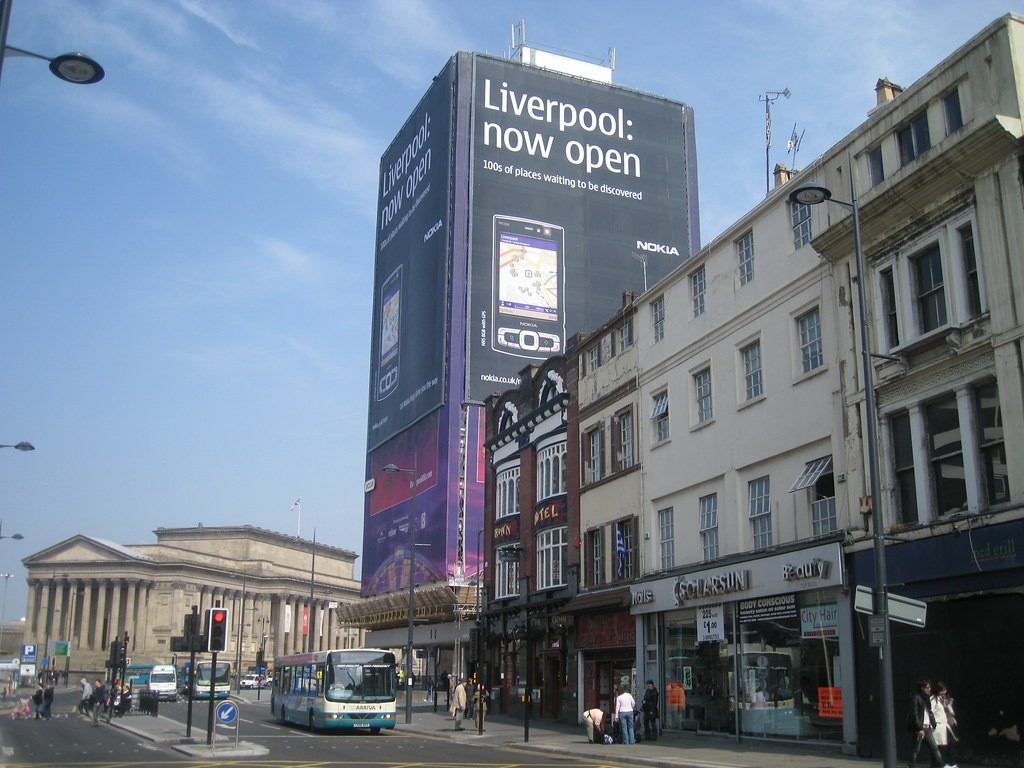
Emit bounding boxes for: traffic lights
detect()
[207,608,230,654]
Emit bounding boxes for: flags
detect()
[617,532,625,576]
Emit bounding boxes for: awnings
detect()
[558,587,632,613]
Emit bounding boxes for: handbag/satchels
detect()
[448,704,456,716]
[945,707,957,728]
[903,711,915,730]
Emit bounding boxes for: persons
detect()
[465,678,474,719]
[42,682,54,721]
[452,679,466,730]
[32,683,44,719]
[752,687,766,710]
[582,708,607,743]
[910,678,958,767]
[615,688,636,744]
[473,683,489,729]
[640,680,660,740]
[78,678,132,727]
[424,677,439,703]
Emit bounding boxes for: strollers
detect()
[7,696,34,721]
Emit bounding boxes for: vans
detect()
[146,664,180,702]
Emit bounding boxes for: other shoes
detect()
[455,727,465,731]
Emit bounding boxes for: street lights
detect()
[63,587,86,689]
[40,568,61,689]
[305,527,334,653]
[236,564,260,694]
[787,152,901,768]
[0,573,16,649]
[381,463,431,723]
[235,590,252,689]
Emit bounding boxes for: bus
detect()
[270,648,405,735]
[179,660,234,699]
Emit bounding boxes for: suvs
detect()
[239,673,267,690]
